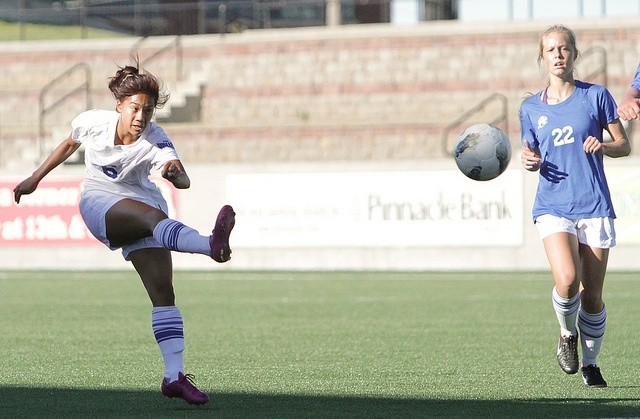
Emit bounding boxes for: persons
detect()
[616,73,639,121]
[14,67,235,404]
[519,27,631,389]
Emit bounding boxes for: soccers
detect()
[454,124,512,181]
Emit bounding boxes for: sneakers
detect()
[557,327,579,374]
[161,371,209,405]
[209,205,235,263]
[581,364,607,387]
